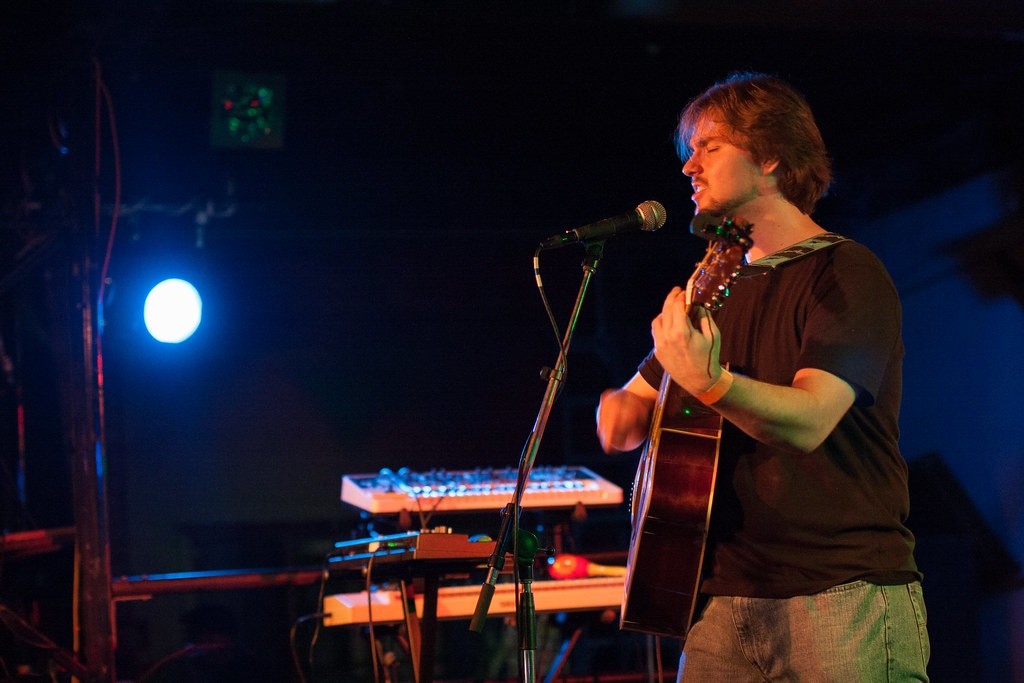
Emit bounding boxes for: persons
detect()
[596,73,935,682]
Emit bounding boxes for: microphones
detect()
[539,201,666,251]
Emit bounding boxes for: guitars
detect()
[620,214,753,641]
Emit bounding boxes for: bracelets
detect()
[696,366,734,407]
